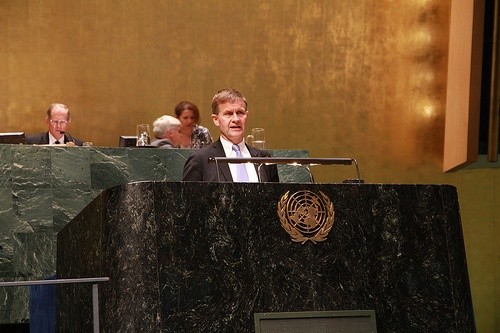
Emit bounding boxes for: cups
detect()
[137,124,151,146]
[252,128,265,149]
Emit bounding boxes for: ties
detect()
[230,145,249,182]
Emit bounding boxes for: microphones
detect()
[60,130,74,142]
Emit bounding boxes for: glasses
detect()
[49,119,69,126]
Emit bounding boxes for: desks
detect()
[0,144,311,325]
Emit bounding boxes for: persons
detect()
[21,103,85,145]
[144,114,182,149]
[182,88,279,182]
[175,101,216,149]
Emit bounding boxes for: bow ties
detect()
[55,141,60,144]
